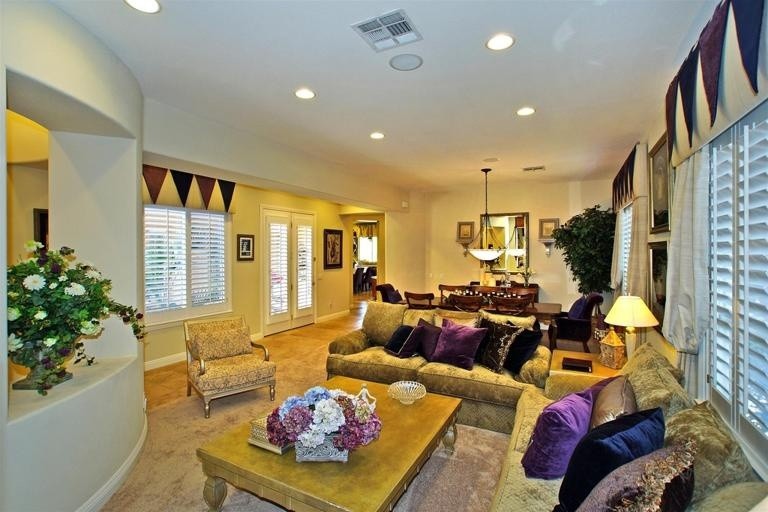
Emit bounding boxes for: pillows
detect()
[520,372,697,512]
[382,315,544,375]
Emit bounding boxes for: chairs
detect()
[353,266,377,294]
[374,284,604,352]
[182,314,277,418]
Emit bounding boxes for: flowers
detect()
[7,239,149,398]
[267,385,382,451]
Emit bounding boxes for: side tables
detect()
[548,348,628,377]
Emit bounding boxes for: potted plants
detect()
[550,203,617,328]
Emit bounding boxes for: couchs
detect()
[489,341,768,512]
[325,300,552,434]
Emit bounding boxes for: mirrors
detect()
[479,212,529,275]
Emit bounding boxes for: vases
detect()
[524,277,529,288]
[11,338,72,390]
[294,436,349,462]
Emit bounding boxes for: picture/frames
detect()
[237,234,254,261]
[324,229,342,270]
[538,218,559,240]
[456,221,474,242]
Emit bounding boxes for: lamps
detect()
[604,295,660,361]
[465,168,506,261]
[504,216,526,257]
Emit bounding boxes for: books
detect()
[562,357,593,373]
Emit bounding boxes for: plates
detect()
[388,381,427,405]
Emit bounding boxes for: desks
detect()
[466,281,538,303]
